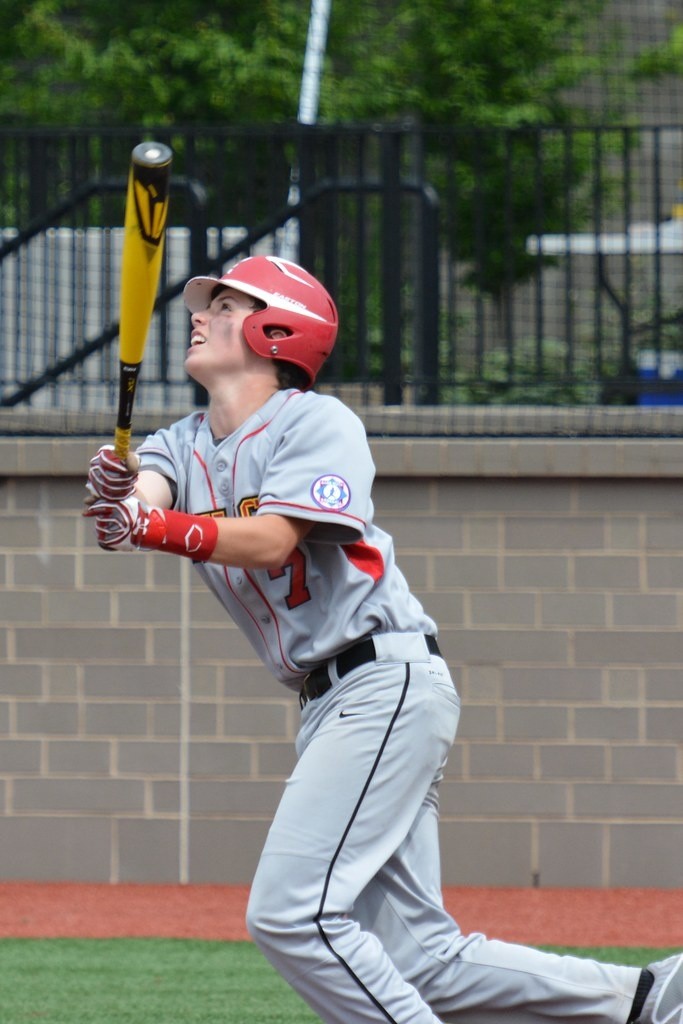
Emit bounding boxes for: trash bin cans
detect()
[635,348,683,406]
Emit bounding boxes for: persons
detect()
[80,254,683,1024]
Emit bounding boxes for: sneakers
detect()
[635,950,683,1024]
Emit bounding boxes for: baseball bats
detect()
[114,141,175,463]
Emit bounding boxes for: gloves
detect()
[81,496,219,563]
[84,444,142,502]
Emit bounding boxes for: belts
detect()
[299,634,444,711]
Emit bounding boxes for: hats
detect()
[181,254,340,392]
